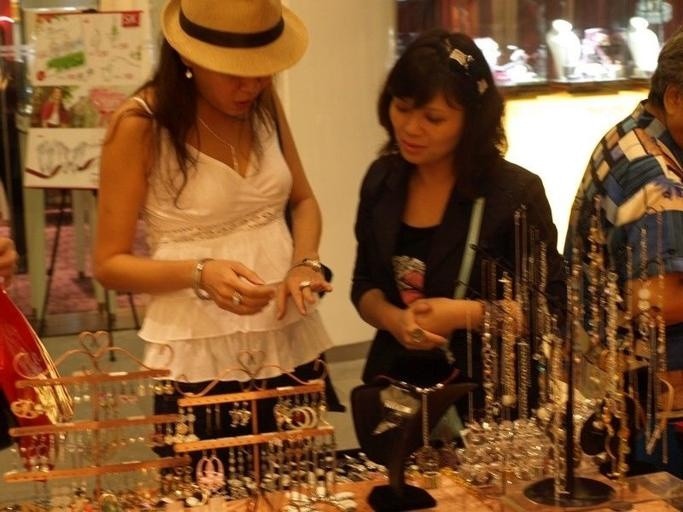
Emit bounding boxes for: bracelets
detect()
[193,258,213,300]
[289,258,321,272]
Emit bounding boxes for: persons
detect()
[1,25,28,275]
[41,89,69,129]
[0,238,20,450]
[350,29,565,449]
[564,32,683,478]
[94,0,346,480]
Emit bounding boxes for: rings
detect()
[230,290,241,306]
[411,328,425,344]
[299,281,310,289]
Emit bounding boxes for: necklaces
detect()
[372,370,457,450]
[482,205,569,497]
[571,217,629,488]
[627,209,674,463]
[195,110,250,173]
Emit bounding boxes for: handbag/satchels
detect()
[0,288,72,475]
[429,194,485,447]
[272,98,346,413]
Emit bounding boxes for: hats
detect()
[160,1,310,78]
